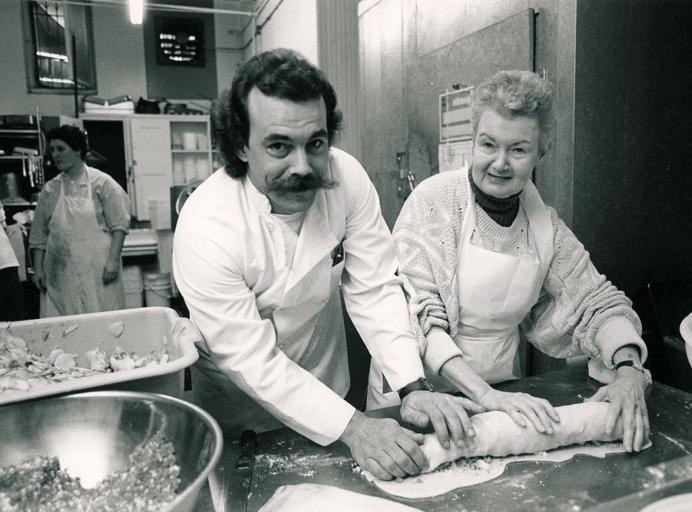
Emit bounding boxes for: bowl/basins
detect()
[0,391,224,512]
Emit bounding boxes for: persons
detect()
[30,125,131,319]
[366,70,653,455]
[172,48,485,481]
[0,200,20,318]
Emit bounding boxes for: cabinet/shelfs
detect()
[58,114,216,227]
[1,105,45,208]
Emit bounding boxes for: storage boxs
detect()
[1,305,201,404]
[121,278,143,309]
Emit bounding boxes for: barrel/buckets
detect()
[142,273,173,307]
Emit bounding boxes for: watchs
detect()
[615,360,644,374]
[397,376,435,400]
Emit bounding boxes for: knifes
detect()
[229,428,257,512]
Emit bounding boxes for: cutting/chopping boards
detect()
[207,362,692,511]
